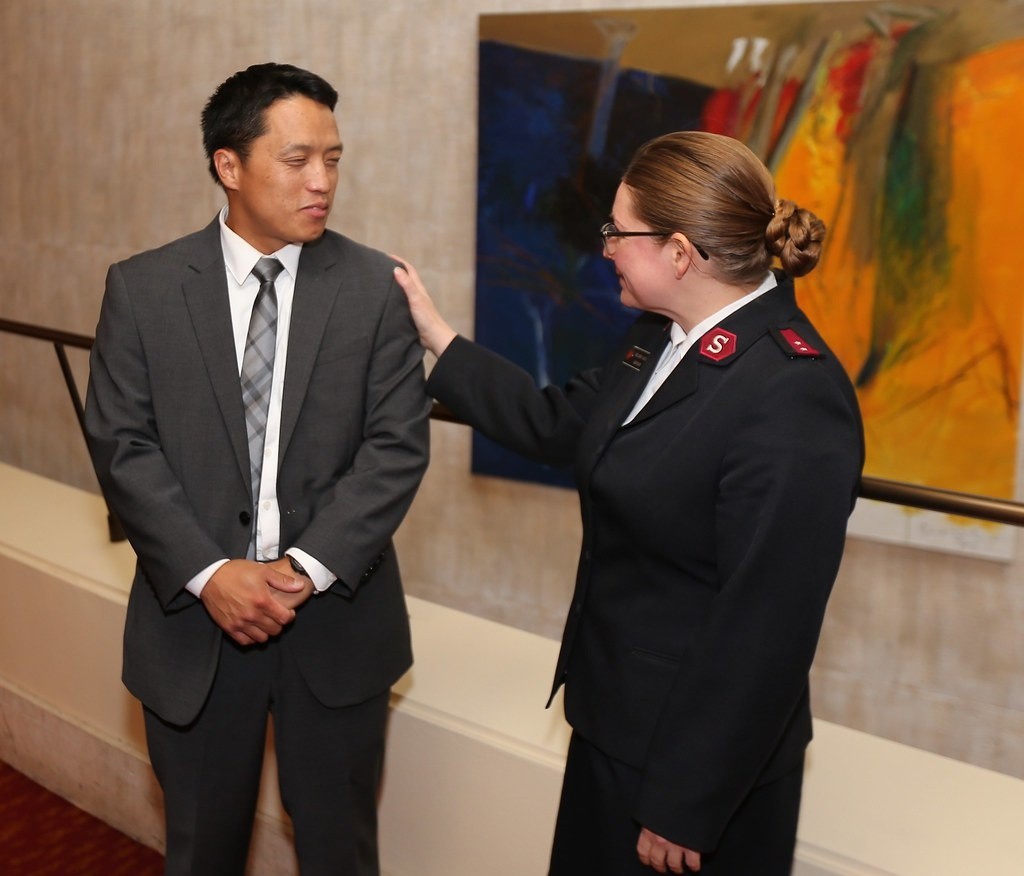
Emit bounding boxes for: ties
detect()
[239,257,286,562]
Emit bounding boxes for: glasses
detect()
[599,223,710,262]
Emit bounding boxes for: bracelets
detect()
[286,554,311,579]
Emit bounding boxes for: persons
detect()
[82,63,433,876]
[389,131,867,876]
